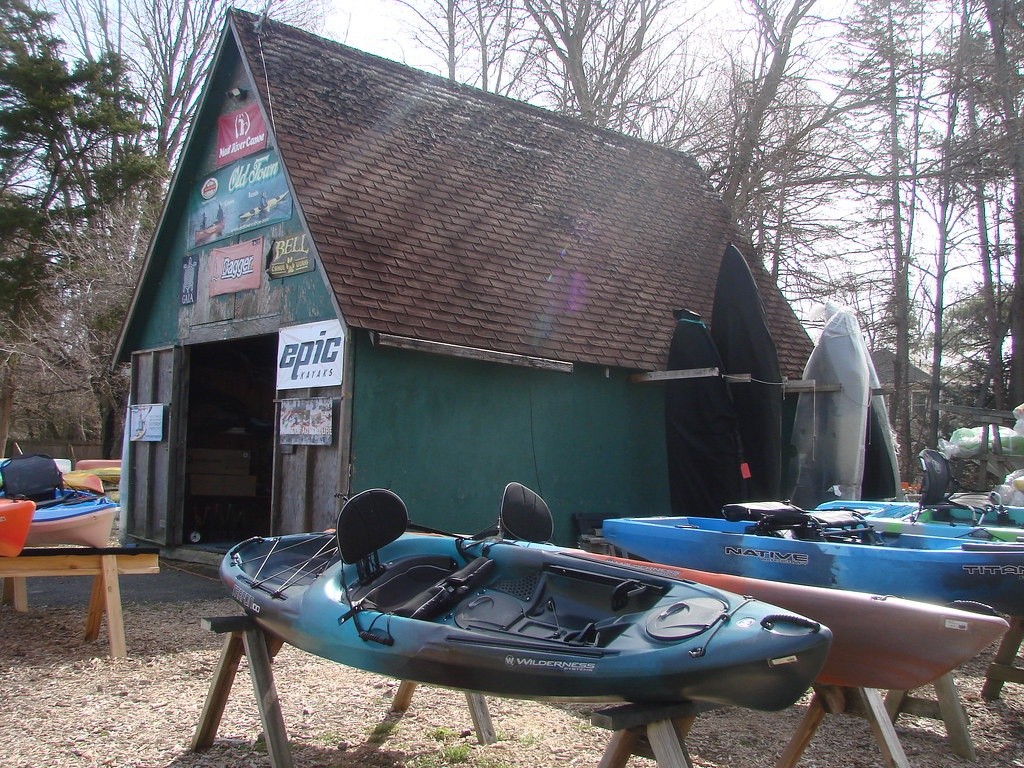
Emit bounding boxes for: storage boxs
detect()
[185,445,257,496]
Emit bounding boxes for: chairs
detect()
[721,502,884,546]
[498,481,553,542]
[901,447,1015,528]
[336,487,494,618]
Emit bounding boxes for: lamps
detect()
[225,87,245,97]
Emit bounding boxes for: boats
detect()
[218,481,834,714]
[0,486,121,549]
[663,306,751,518]
[789,306,905,509]
[601,497,1024,618]
[74,458,121,484]
[708,235,786,505]
[817,446,1023,544]
[60,469,105,495]
[0,494,37,559]
[456,530,1011,692]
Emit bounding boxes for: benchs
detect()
[573,510,629,560]
